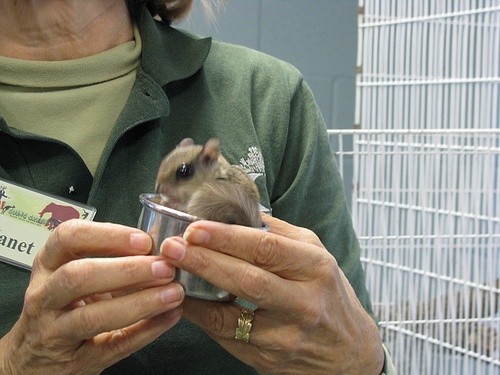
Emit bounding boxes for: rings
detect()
[234,307,258,344]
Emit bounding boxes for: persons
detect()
[0,0,400,375]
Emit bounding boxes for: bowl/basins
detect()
[136,193,271,302]
